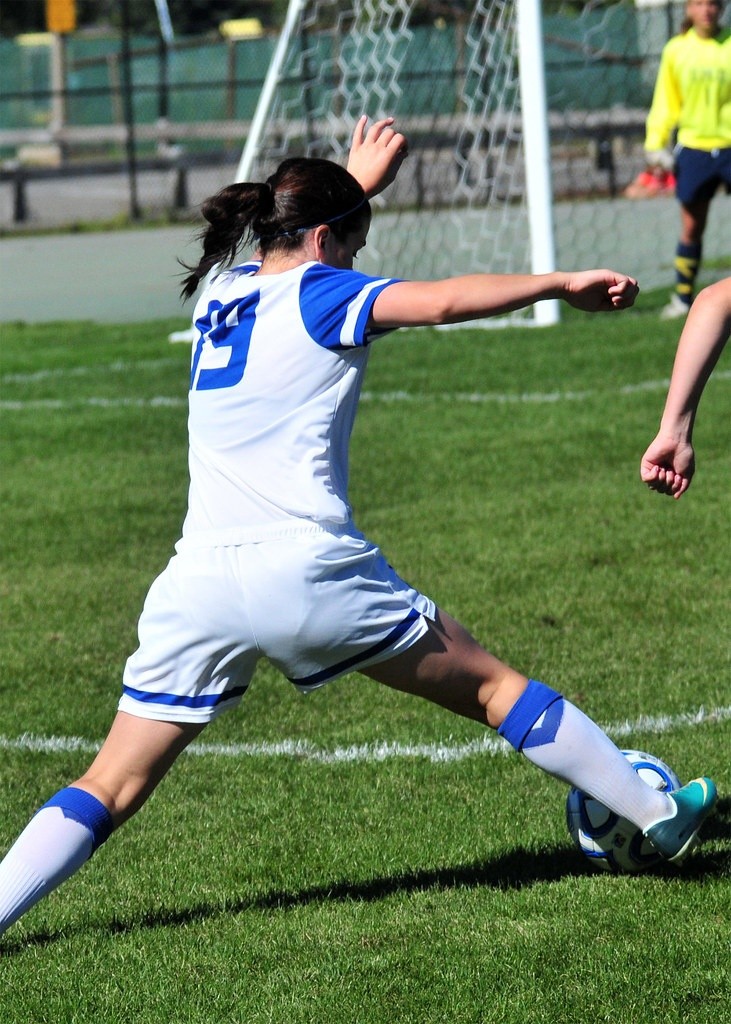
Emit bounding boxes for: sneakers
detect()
[642,776,719,866]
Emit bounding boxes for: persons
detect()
[1,116,717,931]
[640,277,731,499]
[642,0,731,323]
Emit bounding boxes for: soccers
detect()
[563,747,686,873]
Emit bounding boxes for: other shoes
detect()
[661,294,692,321]
